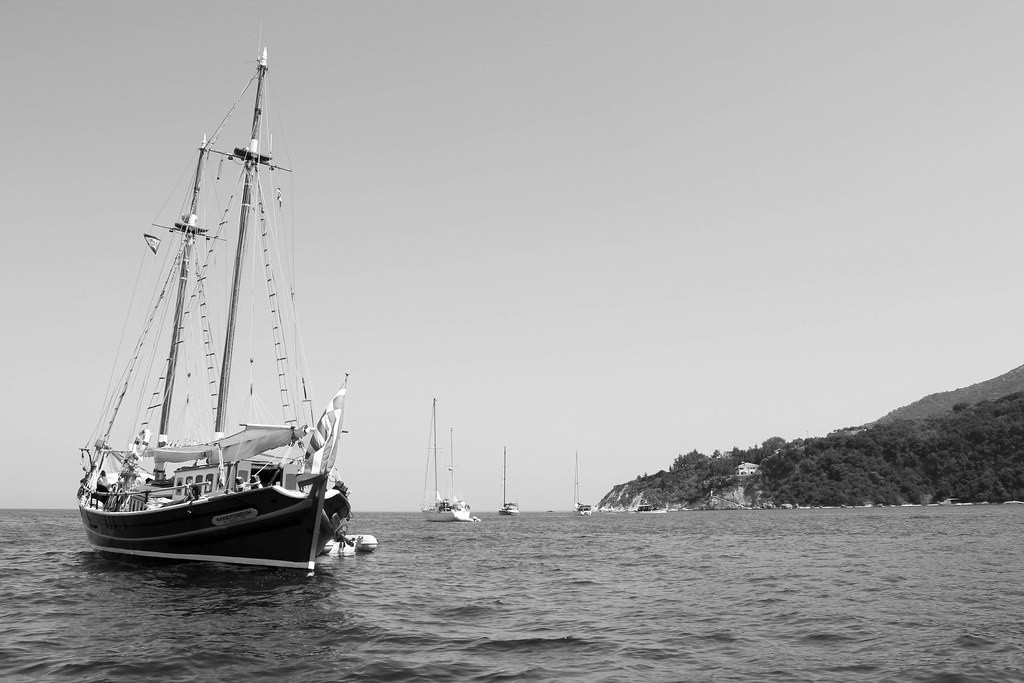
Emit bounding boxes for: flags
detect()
[295,380,345,477]
[144,234,161,254]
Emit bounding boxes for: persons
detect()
[250,475,263,489]
[97,470,109,492]
[235,476,245,491]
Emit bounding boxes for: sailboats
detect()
[421,398,476,524]
[73,52,380,573]
[573,451,598,518]
[498,445,522,516]
